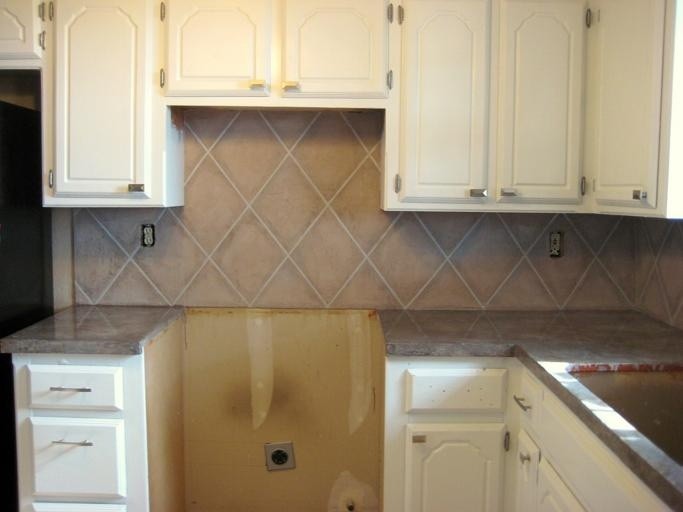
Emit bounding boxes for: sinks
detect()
[565,360,683,465]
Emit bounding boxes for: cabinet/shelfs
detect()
[12,350,184,512]
[164,1,387,108]
[588,1,683,220]
[375,356,515,512]
[382,0,590,213]
[0,1,42,71]
[503,356,670,512]
[40,0,185,208]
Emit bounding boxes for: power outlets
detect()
[141,224,155,246]
[549,232,561,257]
